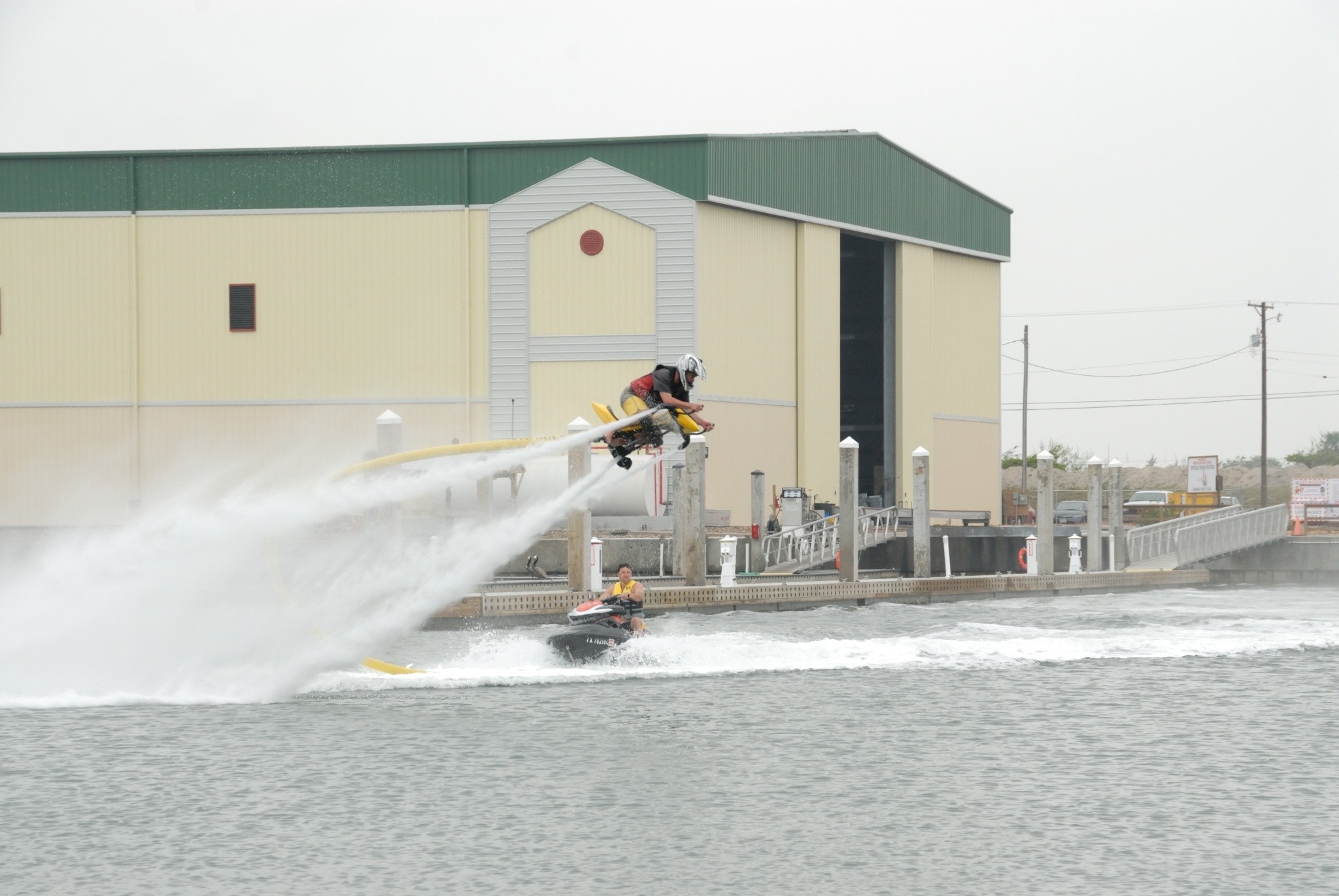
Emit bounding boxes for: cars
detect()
[1220,496,1241,508]
[1053,500,1088,524]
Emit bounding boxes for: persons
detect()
[603,353,714,447]
[594,563,645,636]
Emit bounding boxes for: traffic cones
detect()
[1292,516,1304,536]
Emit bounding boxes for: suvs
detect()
[1124,490,1174,517]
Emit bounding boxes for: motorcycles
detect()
[546,594,648,663]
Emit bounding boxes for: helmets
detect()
[677,354,707,392]
